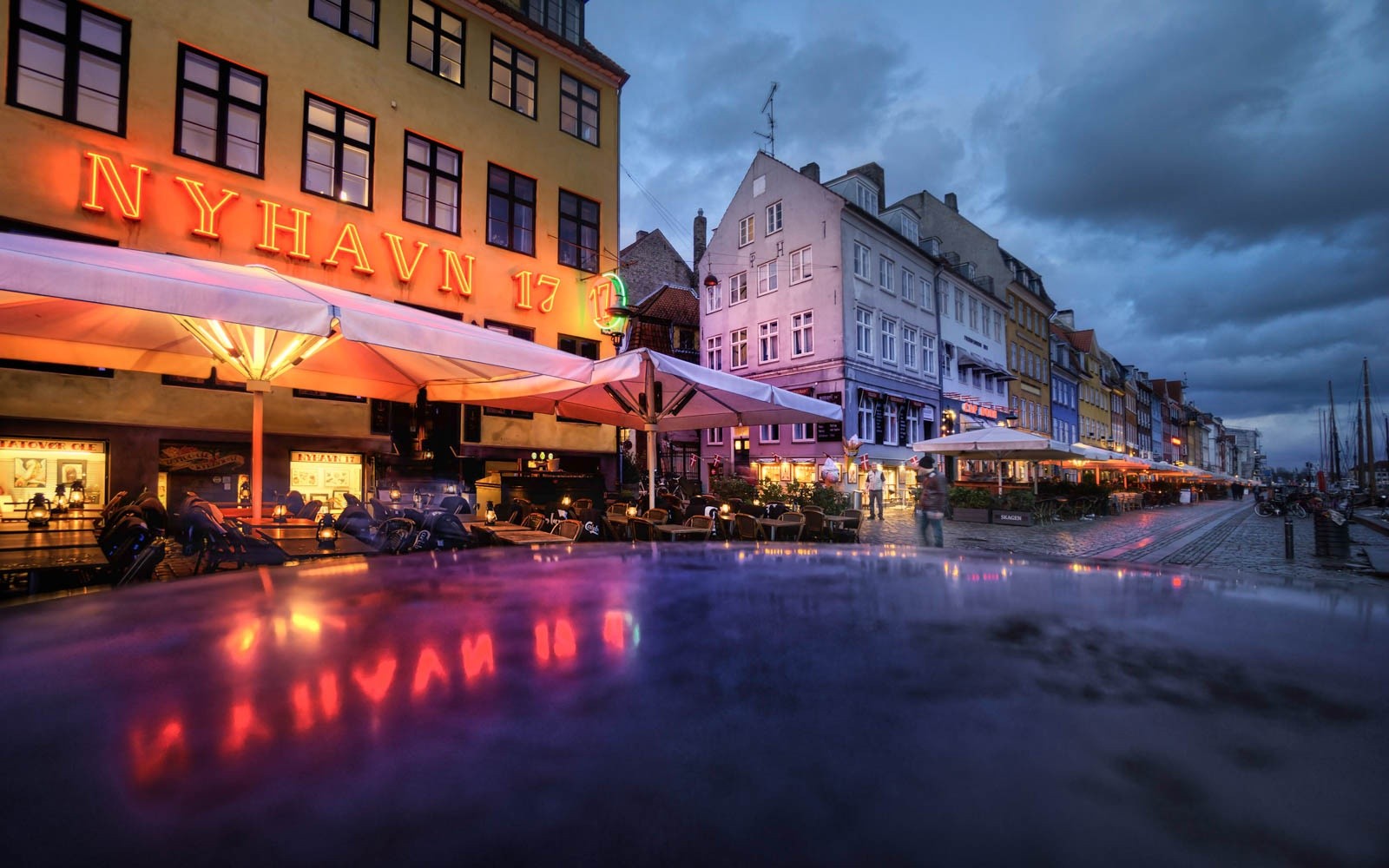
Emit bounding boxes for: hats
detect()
[920,457,935,469]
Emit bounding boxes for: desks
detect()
[0,501,107,599]
[494,529,574,546]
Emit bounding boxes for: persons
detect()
[918,456,947,548]
[1230,481,1244,502]
[865,462,886,520]
[1252,483,1262,502]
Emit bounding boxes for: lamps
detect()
[624,501,639,518]
[315,514,337,547]
[389,483,401,501]
[272,491,288,523]
[25,493,53,526]
[412,488,425,510]
[69,480,86,507]
[239,483,251,503]
[561,493,572,506]
[719,500,731,516]
[703,252,718,287]
[484,500,498,525]
[51,483,68,512]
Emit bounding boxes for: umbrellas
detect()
[1,233,594,523]
[425,347,844,510]
[913,426,1262,499]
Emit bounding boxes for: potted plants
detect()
[993,491,1039,527]
[949,486,992,523]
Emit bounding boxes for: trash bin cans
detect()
[1314,510,1350,556]
[853,490,862,509]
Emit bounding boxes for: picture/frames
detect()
[56,459,87,488]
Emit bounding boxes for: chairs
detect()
[98,490,866,590]
[1035,488,1143,528]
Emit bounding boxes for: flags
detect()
[910,455,920,466]
[772,453,782,465]
[713,454,721,467]
[689,454,697,467]
[860,454,871,472]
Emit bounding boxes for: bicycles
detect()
[1254,492,1306,519]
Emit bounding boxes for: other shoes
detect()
[868,514,876,521]
[878,513,885,520]
